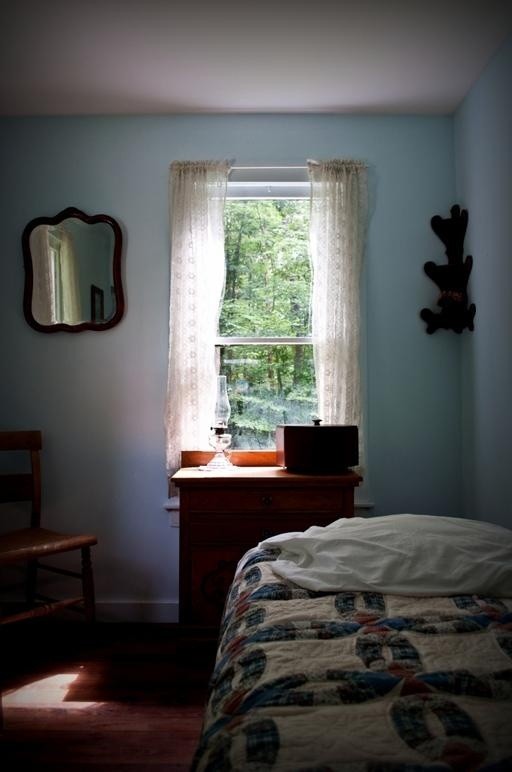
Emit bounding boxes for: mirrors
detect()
[22,204,125,333]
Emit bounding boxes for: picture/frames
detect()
[90,284,104,322]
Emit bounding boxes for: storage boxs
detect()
[275,419,362,474]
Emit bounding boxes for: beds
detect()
[183,510,511,769]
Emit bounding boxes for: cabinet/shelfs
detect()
[172,453,365,640]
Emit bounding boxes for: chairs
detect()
[0,426,98,666]
